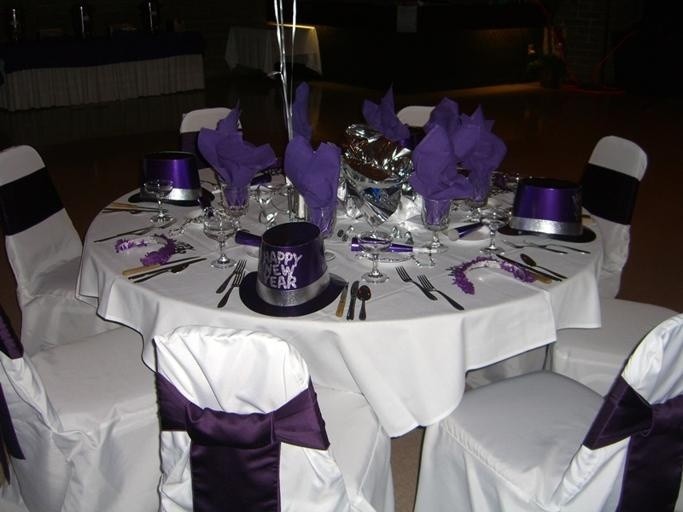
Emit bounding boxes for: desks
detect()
[225,24,323,79]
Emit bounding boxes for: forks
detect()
[217,270,247,309]
[116,219,177,236]
[395,266,438,302]
[417,274,465,311]
[93,225,154,243]
[529,241,592,255]
[501,238,569,254]
[216,261,248,294]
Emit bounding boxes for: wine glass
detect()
[145,152,514,283]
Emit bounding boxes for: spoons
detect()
[357,285,371,321]
[521,252,567,280]
[134,264,190,282]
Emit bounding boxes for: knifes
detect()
[494,254,563,282]
[103,208,167,213]
[128,258,208,280]
[336,282,348,317]
[346,280,360,320]
[122,257,199,277]
[111,202,166,210]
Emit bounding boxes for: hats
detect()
[239,221,346,317]
[496,177,596,243]
[128,150,215,207]
